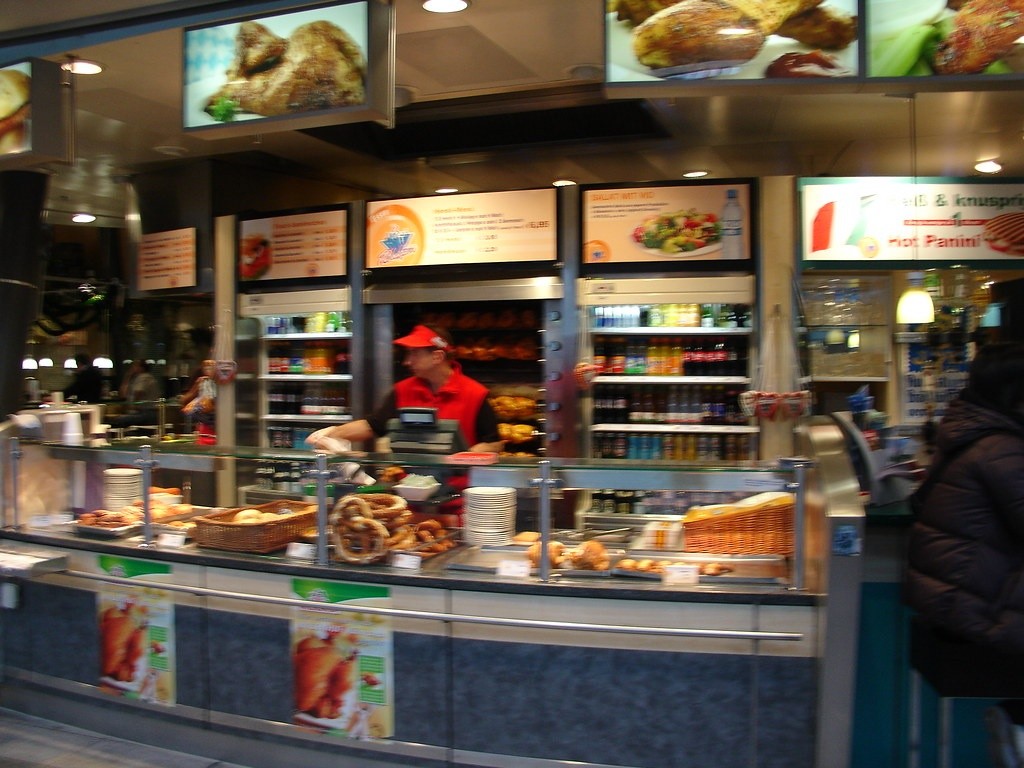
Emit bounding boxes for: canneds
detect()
[273,427,321,452]
[593,431,749,461]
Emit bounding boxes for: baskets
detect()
[685,501,796,558]
[192,500,319,554]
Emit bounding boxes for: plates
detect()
[103,467,144,512]
[630,216,723,257]
[461,486,517,545]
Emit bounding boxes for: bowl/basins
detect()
[298,525,333,543]
[391,483,441,502]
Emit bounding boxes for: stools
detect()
[907,617,1024,768]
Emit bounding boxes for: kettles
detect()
[17,376,51,408]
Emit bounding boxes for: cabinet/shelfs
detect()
[581,325,758,434]
[261,332,356,424]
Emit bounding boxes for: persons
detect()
[63,352,104,404]
[305,323,503,508]
[120,359,160,403]
[901,275,1024,768]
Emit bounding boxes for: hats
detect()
[392,325,452,353]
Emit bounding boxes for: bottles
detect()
[579,490,736,517]
[264,311,351,452]
[722,189,743,259]
[255,461,339,496]
[585,299,760,463]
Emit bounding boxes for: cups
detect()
[62,412,83,446]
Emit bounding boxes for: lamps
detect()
[895,93,936,325]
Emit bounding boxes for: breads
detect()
[80,486,198,528]
[232,492,455,566]
